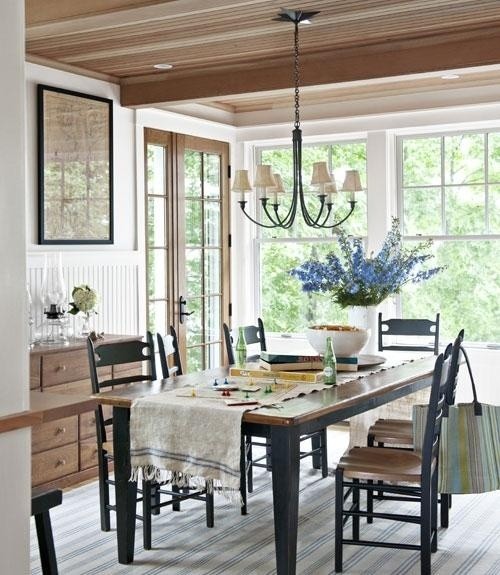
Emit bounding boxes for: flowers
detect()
[286,215,449,309]
[67,285,99,316]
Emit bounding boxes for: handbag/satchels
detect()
[411,345,500,495]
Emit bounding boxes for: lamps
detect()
[232,5,365,231]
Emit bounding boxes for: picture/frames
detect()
[38,83,114,246]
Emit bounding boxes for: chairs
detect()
[367,330,465,529]
[378,312,440,356]
[87,331,214,552]
[157,327,254,515]
[334,343,452,574]
[224,318,328,492]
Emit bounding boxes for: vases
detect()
[81,311,91,336]
[349,306,377,356]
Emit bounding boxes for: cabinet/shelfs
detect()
[29,336,144,495]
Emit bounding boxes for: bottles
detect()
[322,337,337,386]
[25,251,75,347]
[235,326,248,369]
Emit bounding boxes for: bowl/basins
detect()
[306,322,371,357]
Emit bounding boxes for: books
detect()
[229,351,358,383]
